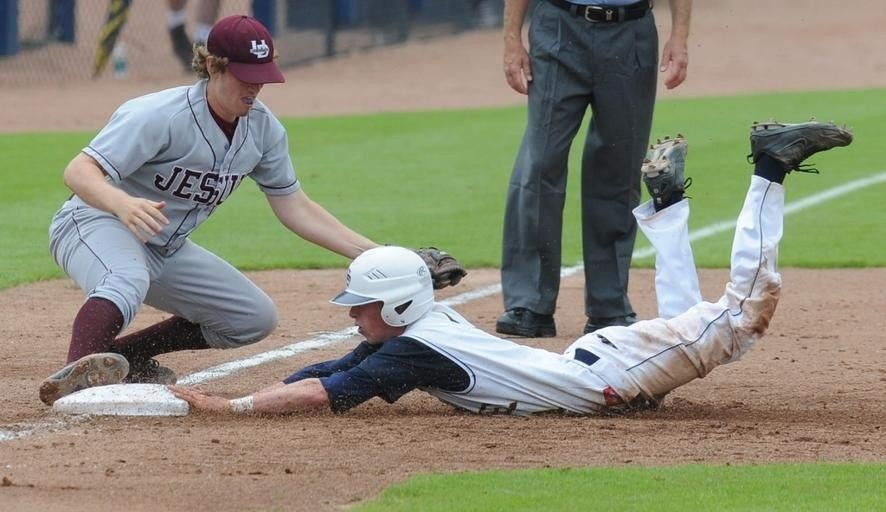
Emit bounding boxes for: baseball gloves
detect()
[409,245,468,293]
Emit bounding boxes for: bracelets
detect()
[228,396,256,416]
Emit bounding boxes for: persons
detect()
[167,1,222,73]
[495,1,693,340]
[166,120,855,418]
[38,14,468,408]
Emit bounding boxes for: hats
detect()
[208,15,286,84]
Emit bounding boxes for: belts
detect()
[555,0,652,25]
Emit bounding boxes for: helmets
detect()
[331,247,435,329]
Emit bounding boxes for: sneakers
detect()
[37,353,129,405]
[641,136,685,196]
[497,308,557,337]
[584,314,636,332]
[749,121,852,167]
[130,360,176,384]
